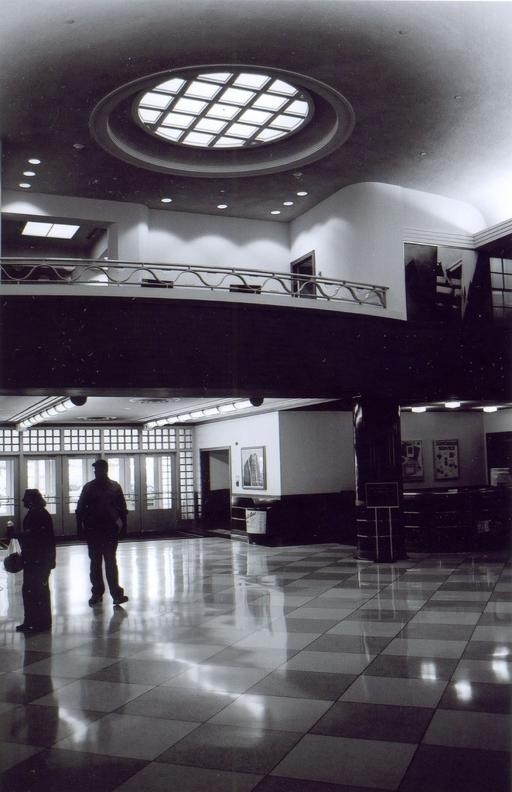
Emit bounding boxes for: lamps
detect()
[14,396,87,434]
[142,398,264,432]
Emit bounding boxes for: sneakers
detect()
[88,595,104,604]
[111,594,129,605]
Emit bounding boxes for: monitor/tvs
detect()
[490,467,512,487]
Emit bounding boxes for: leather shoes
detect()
[15,623,53,633]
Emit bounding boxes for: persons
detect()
[74,459,130,607]
[14,487,58,635]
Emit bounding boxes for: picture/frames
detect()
[400,439,426,484]
[240,444,268,491]
[431,438,461,482]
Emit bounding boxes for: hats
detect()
[92,460,109,467]
